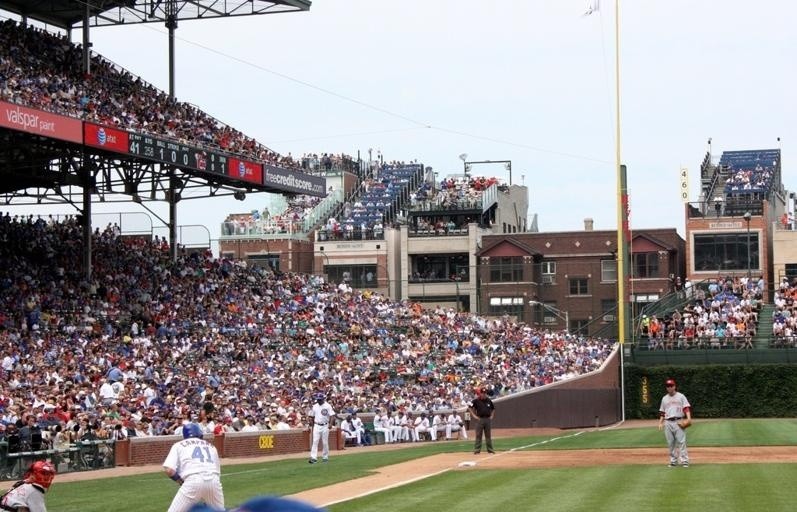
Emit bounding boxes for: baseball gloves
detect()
[678,419,690,429]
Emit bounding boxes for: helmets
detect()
[181,423,204,441]
[22,460,56,490]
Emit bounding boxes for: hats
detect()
[313,393,326,401]
[665,379,676,386]
[480,388,488,394]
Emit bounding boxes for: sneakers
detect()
[308,459,317,464]
[682,464,689,468]
[321,460,328,464]
[667,464,677,468]
[473,451,481,455]
[487,450,495,454]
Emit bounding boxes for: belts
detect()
[667,417,682,421]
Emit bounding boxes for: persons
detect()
[771,277,797,350]
[658,379,692,468]
[714,163,772,219]
[639,275,766,352]
[780,212,797,230]
